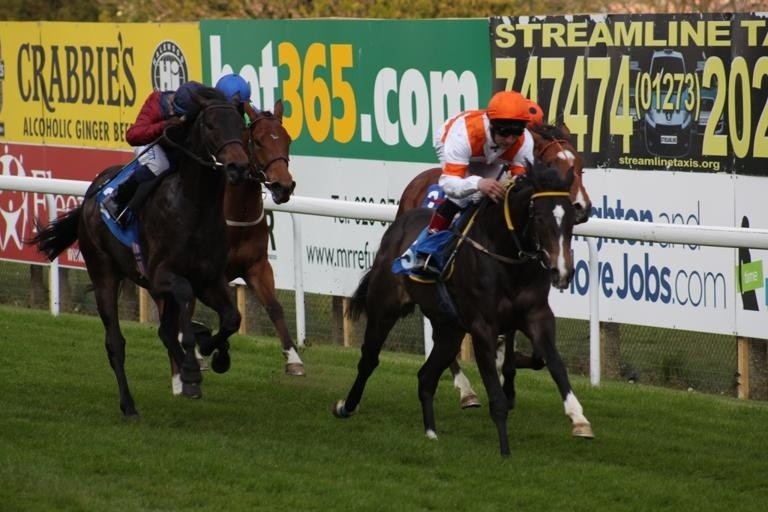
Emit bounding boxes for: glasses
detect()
[493,124,524,136]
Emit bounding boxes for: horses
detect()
[333,158,594,456]
[23,87,250,425]
[85,101,306,397]
[396,122,584,410]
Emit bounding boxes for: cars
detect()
[611,45,726,159]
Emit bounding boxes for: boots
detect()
[102,166,156,229]
[412,211,451,276]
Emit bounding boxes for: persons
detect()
[215,68,252,110]
[98,80,210,234]
[405,88,537,283]
[522,96,549,132]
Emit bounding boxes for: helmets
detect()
[173,80,203,112]
[217,75,251,103]
[487,91,529,124]
[525,99,545,129]
